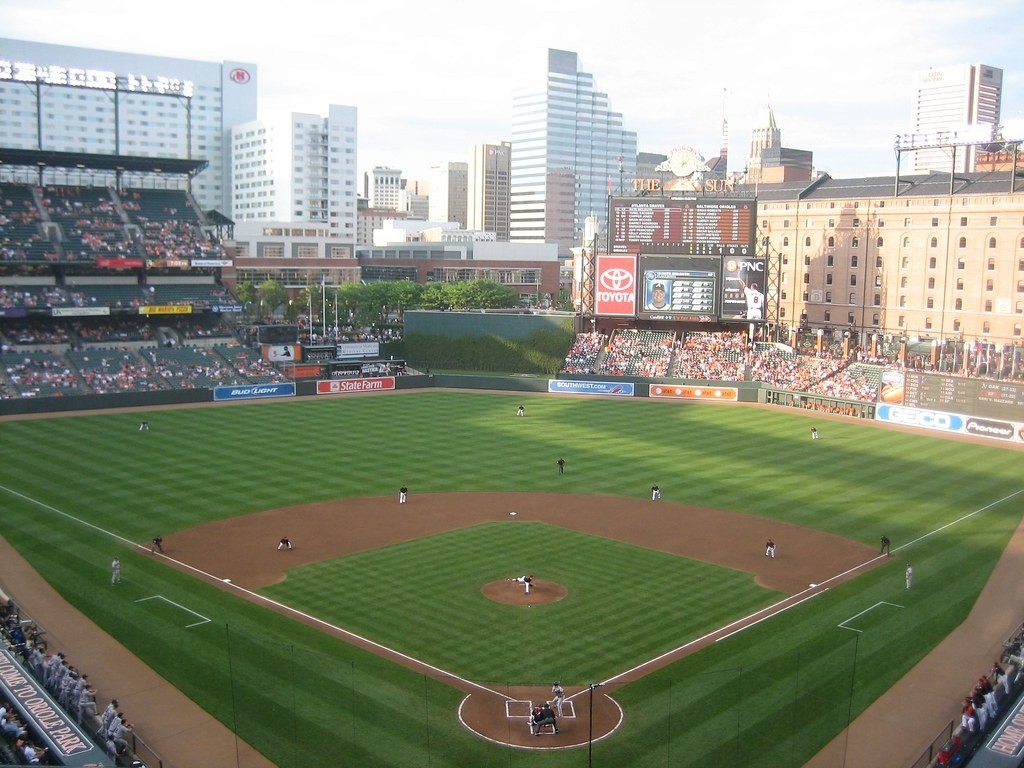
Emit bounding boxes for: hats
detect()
[652,283,664,291]
[113,739,127,747]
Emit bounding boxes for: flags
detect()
[306,280,337,315]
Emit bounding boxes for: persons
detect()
[737,269,763,319]
[651,482,661,501]
[151,534,164,554]
[517,404,524,416]
[277,536,292,550]
[292,317,407,378]
[557,457,565,474]
[765,538,776,558]
[959,625,1024,734]
[138,421,149,430]
[399,484,408,503]
[1,182,291,397]
[516,300,556,314]
[559,331,1024,420]
[526,681,566,736]
[112,556,122,586]
[645,283,671,310]
[879,535,890,558]
[516,575,537,594]
[905,563,913,591]
[810,426,819,439]
[0,597,147,768]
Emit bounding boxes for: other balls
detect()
[528,605,532,608]
